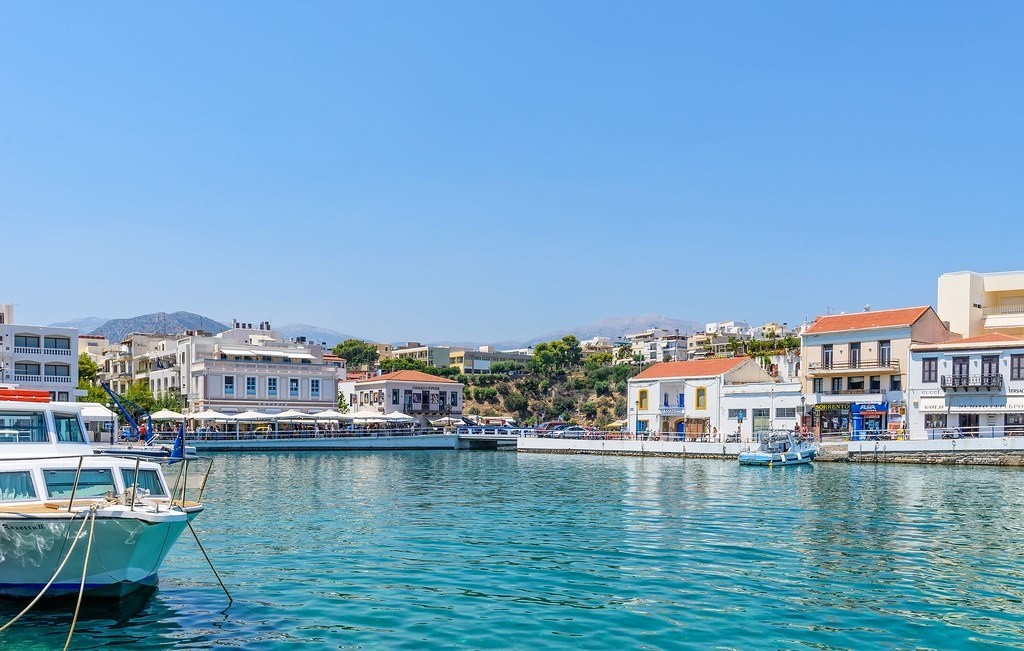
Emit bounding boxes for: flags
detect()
[168,424,183,465]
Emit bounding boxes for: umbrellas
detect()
[142,410,414,419]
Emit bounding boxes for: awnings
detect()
[427,416,467,426]
[919,396,1024,415]
[221,350,317,360]
[604,420,627,428]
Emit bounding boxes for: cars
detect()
[120,432,138,442]
[294,426,319,438]
[553,427,588,438]
[586,427,604,439]
[545,425,568,437]
[339,426,353,431]
[186,428,213,439]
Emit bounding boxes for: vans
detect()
[535,422,564,437]
[252,427,273,434]
[119,426,131,437]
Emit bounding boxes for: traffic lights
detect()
[738,419,742,423]
[738,412,742,419]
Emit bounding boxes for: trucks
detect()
[105,423,112,431]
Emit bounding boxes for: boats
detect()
[0,388,215,596]
[738,429,816,466]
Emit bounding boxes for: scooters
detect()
[942,428,966,440]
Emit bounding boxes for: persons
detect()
[302,422,306,437]
[727,426,740,436]
[801,423,808,438]
[314,423,321,437]
[324,421,329,439]
[139,424,146,440]
[517,414,661,441]
[439,398,443,412]
[330,422,335,438]
[298,423,303,437]
[795,422,800,435]
[712,426,718,442]
[294,423,299,438]
[901,419,907,439]
[342,421,422,436]
[157,423,243,440]
[266,421,274,440]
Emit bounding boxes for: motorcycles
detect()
[725,433,741,443]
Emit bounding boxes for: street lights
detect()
[800,396,805,426]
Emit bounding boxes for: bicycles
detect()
[866,430,892,441]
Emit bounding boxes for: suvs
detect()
[819,417,840,429]
